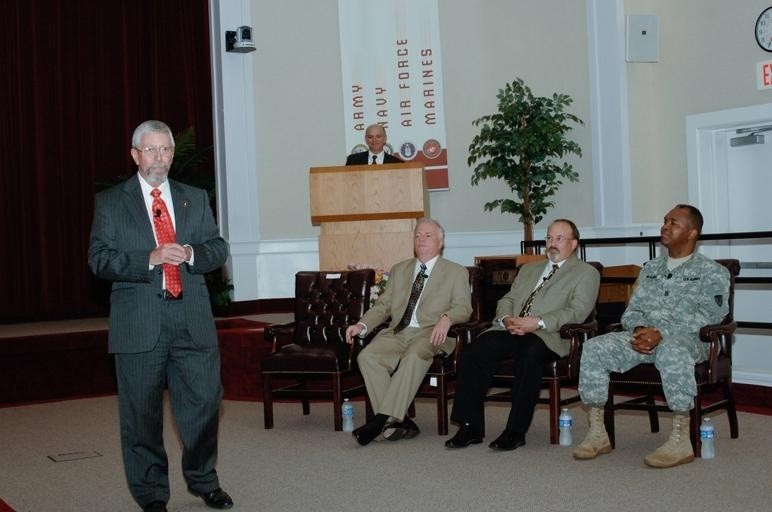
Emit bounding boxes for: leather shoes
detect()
[352,420,381,445]
[488,429,526,451]
[383,416,421,441]
[144,501,168,511]
[187,486,235,510]
[445,428,483,449]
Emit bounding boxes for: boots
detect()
[573,404,612,460]
[644,412,694,468]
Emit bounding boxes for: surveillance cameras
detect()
[232,25,257,50]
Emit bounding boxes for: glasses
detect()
[544,235,574,241]
[134,145,173,156]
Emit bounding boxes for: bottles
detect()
[559,407,572,444]
[342,398,353,432]
[701,418,715,458]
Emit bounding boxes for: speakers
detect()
[626,13,660,64]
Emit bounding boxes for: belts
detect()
[160,289,183,300]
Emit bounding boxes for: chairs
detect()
[261,268,375,431]
[365,266,476,436]
[481,262,604,447]
[603,258,739,458]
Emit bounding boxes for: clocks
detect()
[754,7,772,52]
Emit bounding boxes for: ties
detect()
[393,264,427,335]
[520,264,560,317]
[151,189,183,298]
[372,156,377,164]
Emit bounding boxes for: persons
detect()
[443,219,600,451]
[345,220,473,445]
[571,205,731,468]
[87,120,234,512]
[345,124,404,165]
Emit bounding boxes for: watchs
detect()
[537,315,543,329]
